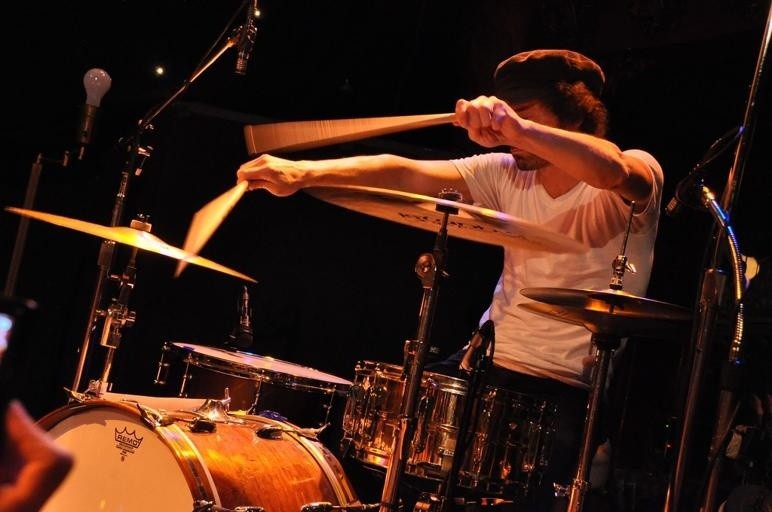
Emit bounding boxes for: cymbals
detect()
[7,207,259,283]
[516,285,677,333]
[306,184,591,257]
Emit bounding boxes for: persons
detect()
[0,390,76,510]
[232,47,667,511]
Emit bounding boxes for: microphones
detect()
[235,0,258,76]
[238,286,254,349]
[665,144,716,219]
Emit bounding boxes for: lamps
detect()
[79,68,113,159]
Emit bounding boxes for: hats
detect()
[492,47,608,99]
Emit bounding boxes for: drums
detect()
[30,392,366,511]
[155,340,354,446]
[340,358,554,499]
